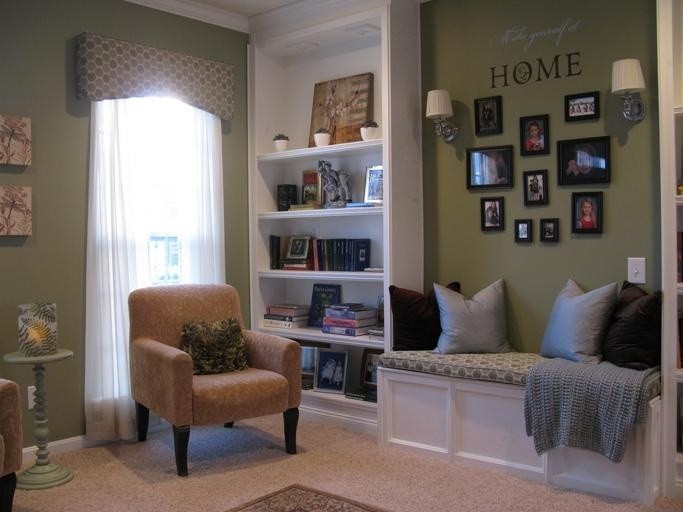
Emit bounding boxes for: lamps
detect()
[610,58,646,124]
[426,89,459,144]
[17,303,59,356]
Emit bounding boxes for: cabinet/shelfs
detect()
[248,1,423,437]
[673,108,683,490]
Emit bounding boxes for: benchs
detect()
[377,351,661,505]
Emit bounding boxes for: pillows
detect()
[389,278,662,372]
[181,316,250,376]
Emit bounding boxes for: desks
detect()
[3,350,72,488]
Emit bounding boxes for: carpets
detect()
[225,483,389,512]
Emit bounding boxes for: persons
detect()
[578,196,597,231]
[317,159,346,206]
[543,226,552,235]
[571,102,594,114]
[365,360,374,383]
[525,122,543,152]
[566,143,598,176]
[320,353,343,387]
[482,102,494,127]
[485,201,499,225]
[529,176,543,201]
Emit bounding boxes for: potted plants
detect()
[272,131,289,150]
[314,128,330,147]
[359,120,378,141]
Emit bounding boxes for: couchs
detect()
[128,283,302,477]
[0,378,22,512]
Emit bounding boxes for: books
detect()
[346,203,375,208]
[278,258,313,271]
[262,303,311,328]
[269,233,281,270]
[309,238,384,272]
[321,303,383,336]
[346,387,378,403]
[287,202,319,211]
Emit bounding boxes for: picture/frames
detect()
[286,233,311,259]
[309,73,372,147]
[465,91,611,244]
[364,166,384,205]
[315,348,350,394]
[360,348,384,389]
[302,170,324,208]
